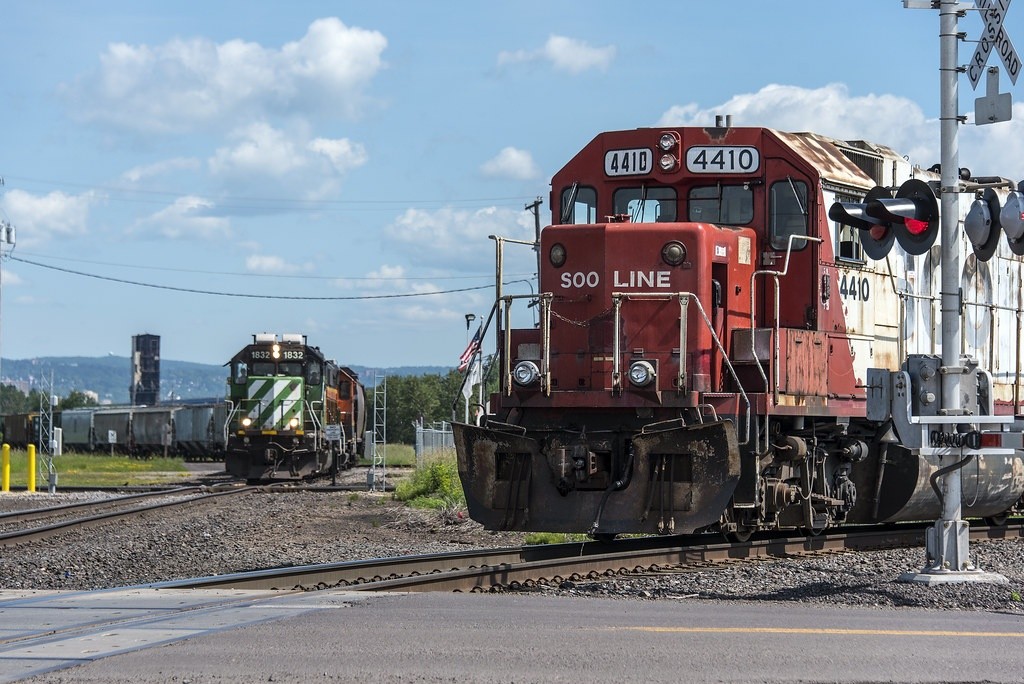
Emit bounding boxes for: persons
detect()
[236,367,320,385]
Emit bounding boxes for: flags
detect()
[458,325,482,400]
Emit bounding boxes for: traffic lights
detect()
[828,178,939,261]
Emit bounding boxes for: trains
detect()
[450,112,1024,548]
[0,332,366,487]
